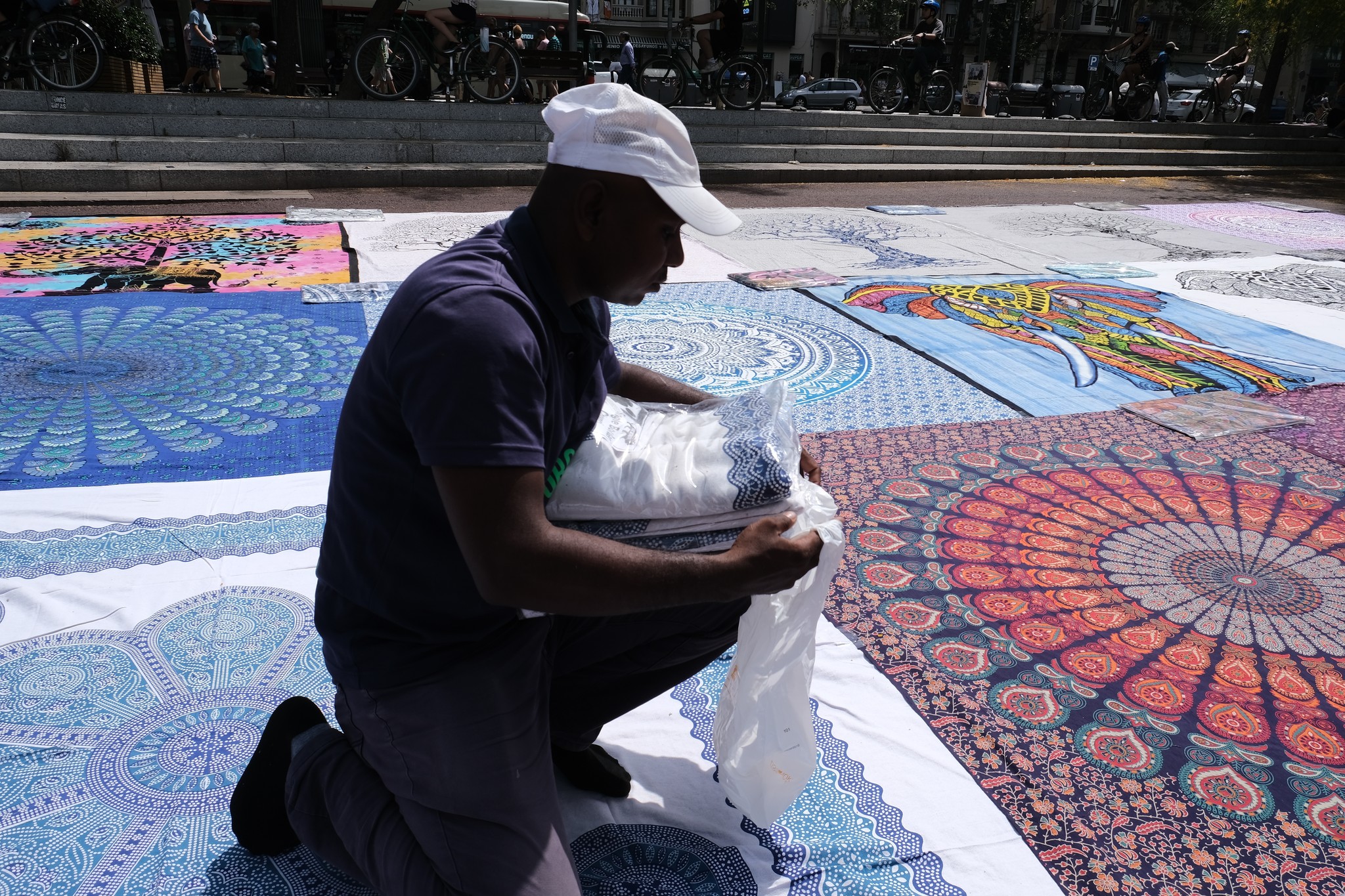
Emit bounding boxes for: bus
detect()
[319,0,609,99]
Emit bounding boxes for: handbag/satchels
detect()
[706,477,848,827]
[862,84,867,92]
[795,79,799,87]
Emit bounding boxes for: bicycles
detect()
[1081,50,1155,122]
[351,0,523,105]
[0,0,107,92]
[296,77,321,97]
[635,20,767,111]
[1304,108,1332,125]
[1192,63,1245,124]
[866,36,957,116]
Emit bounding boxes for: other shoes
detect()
[1315,119,1321,123]
[1159,118,1170,122]
[865,101,868,105]
[1327,134,1345,138]
[508,98,551,105]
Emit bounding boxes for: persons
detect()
[677,0,744,105]
[371,36,404,94]
[602,31,638,93]
[858,76,868,105]
[1206,30,1252,109]
[1032,80,1071,119]
[1305,92,1330,123]
[798,70,812,88]
[889,0,946,112]
[241,22,276,93]
[1103,14,1180,122]
[1326,82,1345,138]
[323,48,350,97]
[1275,91,1284,106]
[483,15,561,105]
[178,0,226,96]
[231,82,823,896]
[425,0,477,94]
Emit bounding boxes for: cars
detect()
[885,85,963,115]
[1165,89,1256,124]
[775,77,864,111]
[1249,97,1297,124]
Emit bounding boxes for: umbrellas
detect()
[140,0,164,50]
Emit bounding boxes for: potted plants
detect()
[68,0,165,93]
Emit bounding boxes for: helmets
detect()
[1237,30,1252,36]
[919,0,940,17]
[1136,16,1150,27]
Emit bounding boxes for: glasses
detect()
[200,0,210,5]
[251,29,260,33]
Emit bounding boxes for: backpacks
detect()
[1314,95,1326,104]
[1144,52,1169,79]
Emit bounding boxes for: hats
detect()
[195,0,210,4]
[808,76,815,81]
[1166,41,1179,50]
[542,80,742,236]
[260,41,277,50]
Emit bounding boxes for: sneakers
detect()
[761,96,770,102]
[901,98,916,112]
[431,82,457,94]
[699,61,720,74]
[178,82,227,94]
[1220,102,1228,108]
[922,76,931,85]
[371,85,379,92]
[1128,89,1136,97]
[1103,107,1115,113]
[444,41,466,55]
[696,89,718,103]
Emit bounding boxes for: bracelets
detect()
[632,68,635,70]
[923,32,926,37]
[893,39,896,44]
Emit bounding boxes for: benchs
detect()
[463,48,589,101]
[995,90,1055,119]
[295,68,347,96]
